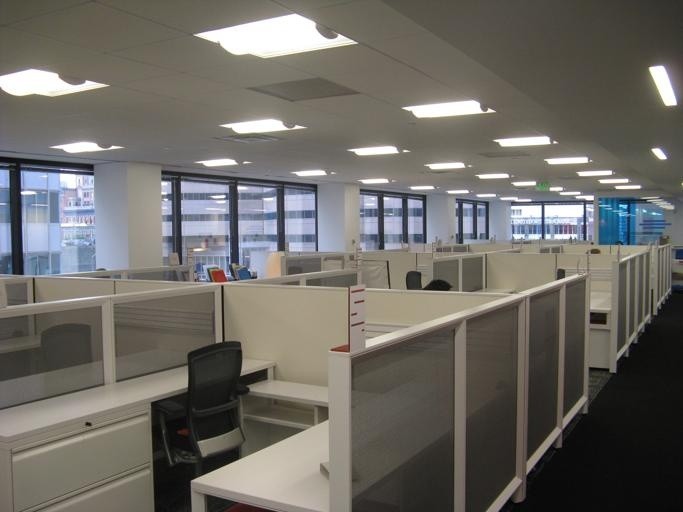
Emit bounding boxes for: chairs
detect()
[406,271,452,291]
[153,341,247,510]
[37,322,93,370]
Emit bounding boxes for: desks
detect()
[191,419,331,511]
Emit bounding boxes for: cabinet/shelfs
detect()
[242,380,383,430]
[589,292,612,368]
[13,413,152,510]
